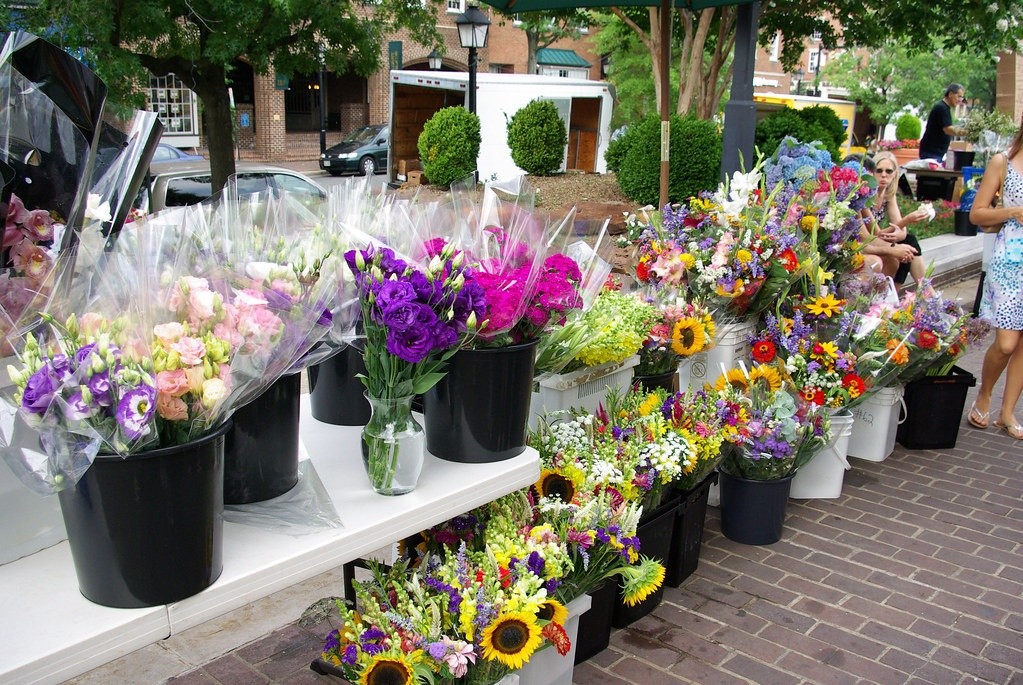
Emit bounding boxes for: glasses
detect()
[876,169,895,174]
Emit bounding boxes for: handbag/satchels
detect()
[979,151,1007,233]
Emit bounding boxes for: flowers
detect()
[0,140,991,684]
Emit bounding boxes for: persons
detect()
[969,110,1023,440]
[972,192,998,318]
[956,98,969,117]
[842,152,919,278]
[871,151,936,297]
[919,83,969,162]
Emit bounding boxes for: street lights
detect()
[813,41,847,98]
[313,39,327,154]
[452,5,492,184]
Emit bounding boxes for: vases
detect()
[895,362,977,450]
[310,323,372,428]
[953,210,976,237]
[703,320,758,384]
[614,493,686,630]
[573,555,619,663]
[789,407,853,500]
[952,149,976,169]
[716,464,797,547]
[509,590,591,685]
[665,470,719,589]
[46,413,233,610]
[632,369,677,400]
[525,358,641,435]
[223,370,302,507]
[846,386,909,463]
[359,388,425,496]
[423,335,542,464]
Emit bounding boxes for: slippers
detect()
[968,400,989,428]
[993,420,1023,440]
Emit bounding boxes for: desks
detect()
[906,168,963,202]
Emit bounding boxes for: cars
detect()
[318,123,389,176]
[133,161,333,216]
[149,142,205,166]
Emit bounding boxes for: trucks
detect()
[385,67,617,190]
[754,90,873,162]
